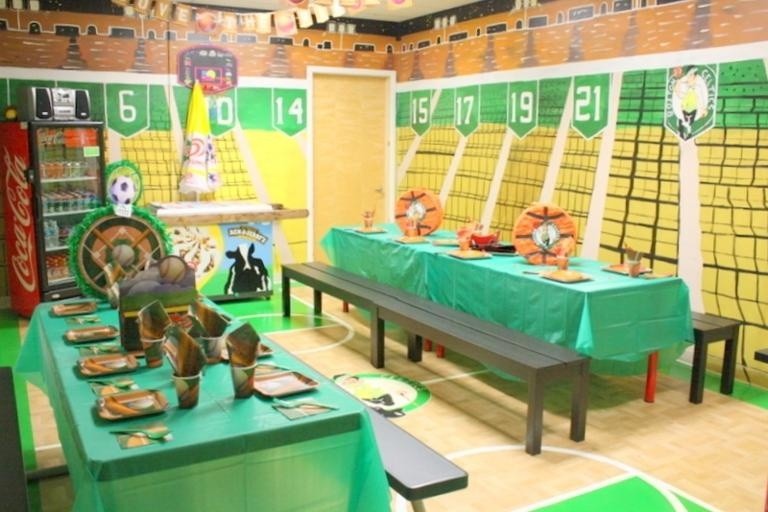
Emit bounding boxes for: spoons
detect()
[109,428,173,440]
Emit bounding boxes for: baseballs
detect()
[161,257,185,283]
[111,246,135,267]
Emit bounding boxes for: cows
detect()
[223,243,272,297]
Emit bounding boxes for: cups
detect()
[556,256,570,272]
[628,259,640,277]
[362,214,374,229]
[230,363,257,399]
[138,336,167,367]
[172,371,203,407]
[198,335,225,364]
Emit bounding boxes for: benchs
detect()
[0,366,29,512]
[679,311,745,406]
[286,261,421,367]
[282,350,470,512]
[377,290,590,455]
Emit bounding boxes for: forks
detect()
[273,397,341,412]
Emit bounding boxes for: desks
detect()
[331,220,684,403]
[35,292,362,512]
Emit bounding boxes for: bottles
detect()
[43,220,60,252]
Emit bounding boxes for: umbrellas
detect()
[176,77,222,205]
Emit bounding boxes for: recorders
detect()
[15,86,91,122]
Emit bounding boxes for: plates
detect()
[541,268,588,283]
[605,261,652,276]
[354,227,520,259]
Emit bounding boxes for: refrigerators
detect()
[148,201,273,303]
[1,120,110,320]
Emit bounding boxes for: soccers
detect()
[111,174,136,203]
